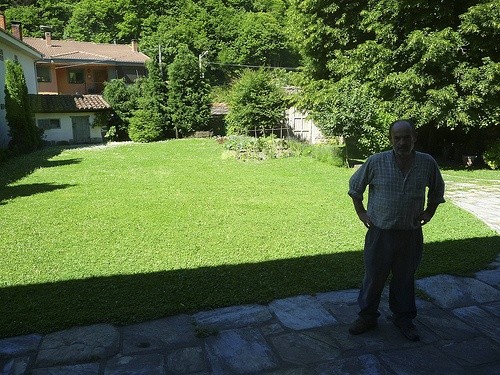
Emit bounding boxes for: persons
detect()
[347,119,445,340]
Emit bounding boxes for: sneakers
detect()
[350,315,377,335]
[392,313,422,341]
